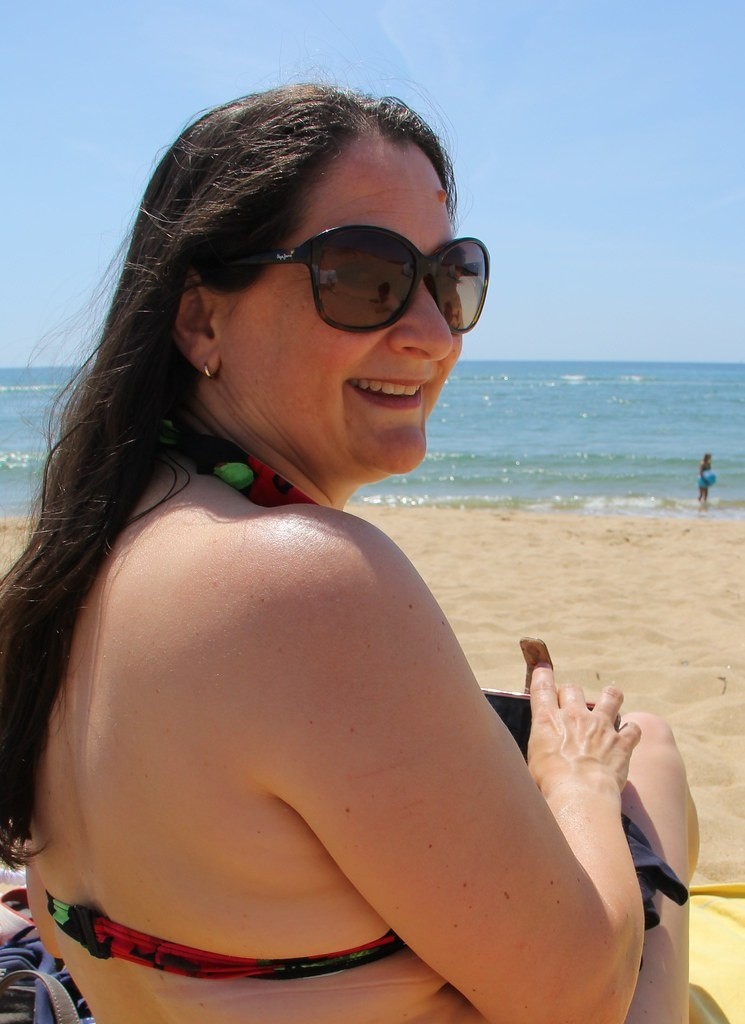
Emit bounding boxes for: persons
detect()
[379,280,390,303]
[444,302,463,329]
[698,453,711,501]
[329,266,337,290]
[0,85,699,1024]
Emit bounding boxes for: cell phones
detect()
[478,688,621,767]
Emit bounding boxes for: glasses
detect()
[264,224,491,335]
